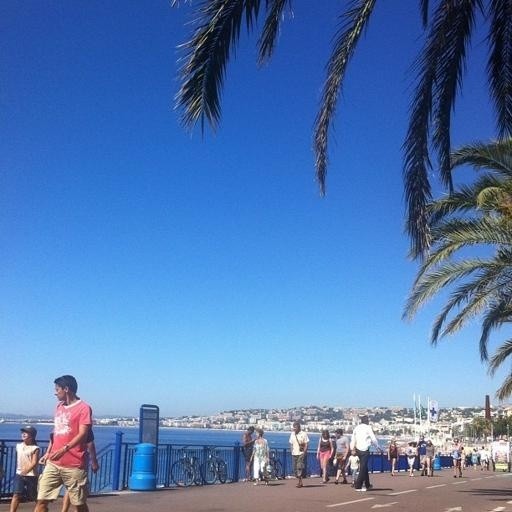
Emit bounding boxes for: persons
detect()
[10,425,41,512]
[388,438,399,477]
[405,435,489,477]
[33,374,92,512]
[349,416,379,492]
[345,448,361,488]
[242,426,255,482]
[316,429,350,485]
[38,430,99,512]
[249,429,270,486]
[288,423,310,488]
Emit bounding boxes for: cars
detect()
[494,460,508,473]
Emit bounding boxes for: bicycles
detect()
[171,444,202,489]
[198,445,229,484]
[264,449,283,482]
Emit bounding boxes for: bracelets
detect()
[64,444,68,451]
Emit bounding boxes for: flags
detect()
[413,392,438,422]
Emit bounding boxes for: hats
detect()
[20,425,37,437]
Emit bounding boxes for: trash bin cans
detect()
[128,442,158,491]
[432,457,441,470]
